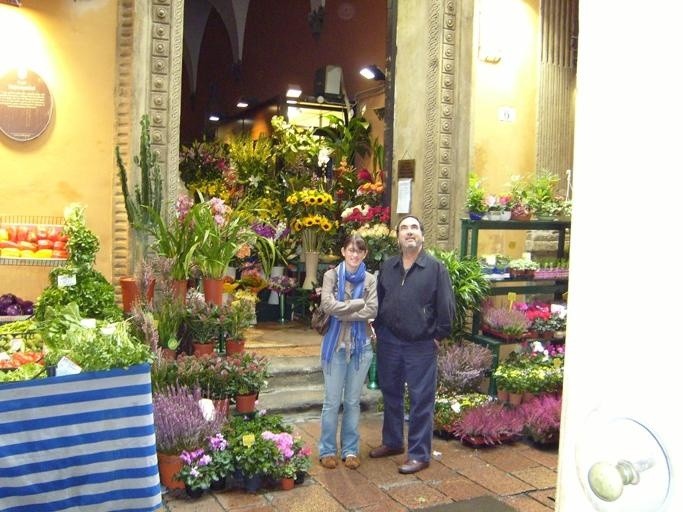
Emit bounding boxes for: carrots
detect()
[1,351,44,370]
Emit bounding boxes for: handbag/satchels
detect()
[312,307,331,335]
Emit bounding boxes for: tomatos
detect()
[0,226,69,259]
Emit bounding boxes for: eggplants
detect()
[0,293,34,316]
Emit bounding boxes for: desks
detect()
[0,365,165,511]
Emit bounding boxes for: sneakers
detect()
[320,456,337,469]
[398,459,428,474]
[370,445,404,458]
[345,455,360,469]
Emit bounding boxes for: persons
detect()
[368,214,456,473]
[318,232,379,469]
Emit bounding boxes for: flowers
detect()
[131,115,572,493]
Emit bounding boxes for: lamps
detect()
[205,105,223,123]
[233,96,251,113]
[279,80,305,103]
[359,63,385,81]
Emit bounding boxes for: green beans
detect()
[0,318,33,333]
